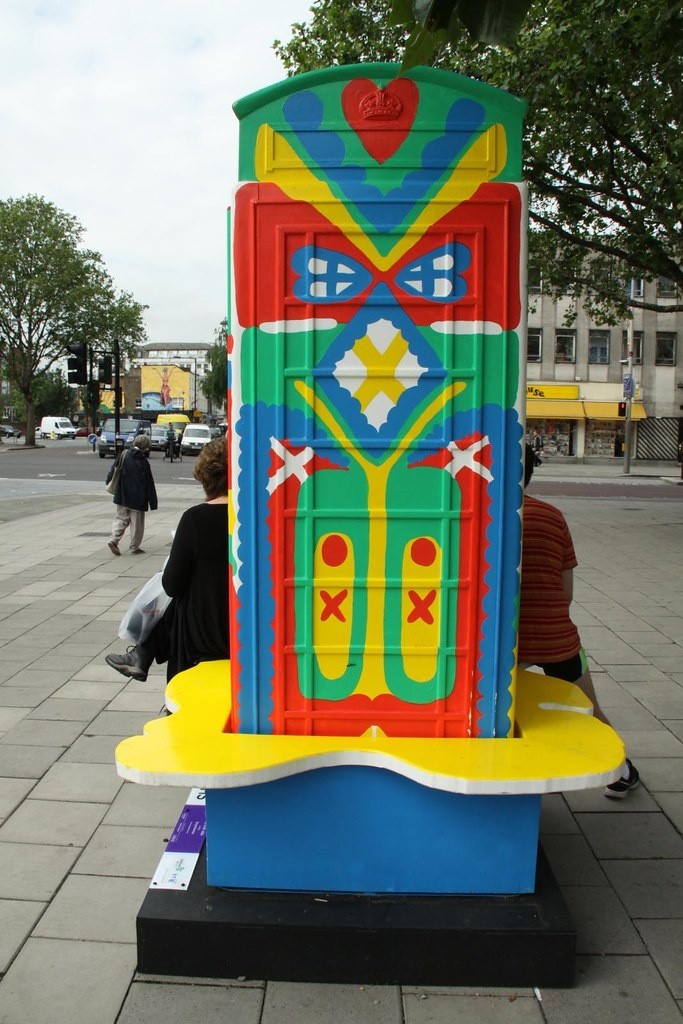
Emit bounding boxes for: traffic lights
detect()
[87,380,100,405]
[67,340,88,385]
[617,402,626,416]
[98,354,113,386]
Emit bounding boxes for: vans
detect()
[40,416,76,440]
[98,417,152,457]
[156,414,190,434]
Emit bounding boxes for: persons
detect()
[181,423,187,434]
[151,367,178,407]
[532,429,544,466]
[106,437,231,715]
[107,435,158,555]
[164,422,178,458]
[518,443,639,799]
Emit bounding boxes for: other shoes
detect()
[130,548,145,554]
[108,541,121,556]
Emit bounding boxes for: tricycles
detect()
[162,439,183,463]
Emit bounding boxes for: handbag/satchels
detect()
[106,450,128,495]
[118,556,174,645]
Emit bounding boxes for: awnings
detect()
[525,401,646,422]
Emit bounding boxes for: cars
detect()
[181,423,212,455]
[75,427,91,437]
[209,424,226,438]
[0,424,22,438]
[151,424,168,451]
[35,426,58,440]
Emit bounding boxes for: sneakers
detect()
[105,646,155,682]
[604,758,639,799]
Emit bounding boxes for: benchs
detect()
[115,658,629,897]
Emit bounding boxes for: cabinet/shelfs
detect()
[526,422,571,456]
[585,420,616,457]
